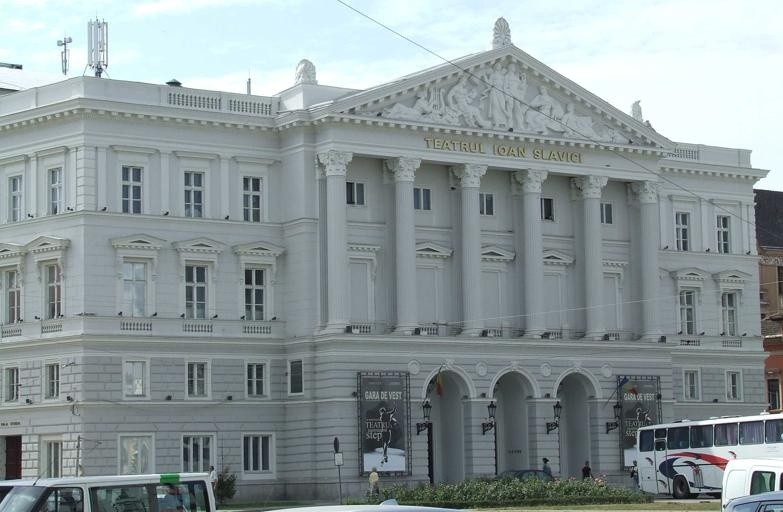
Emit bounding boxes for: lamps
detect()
[481,401,497,436]
[606,400,623,434]
[546,400,562,434]
[417,400,432,434]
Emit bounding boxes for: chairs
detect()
[752,471,775,495]
[59,494,149,512]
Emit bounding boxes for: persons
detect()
[630,459,640,489]
[368,467,379,492]
[377,403,398,463]
[582,460,593,480]
[542,455,551,477]
[208,465,218,489]
[635,408,653,425]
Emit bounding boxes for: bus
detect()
[637,410,783,499]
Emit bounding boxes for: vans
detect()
[0,473,216,512]
[721,458,783,512]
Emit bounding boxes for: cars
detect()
[724,490,783,512]
